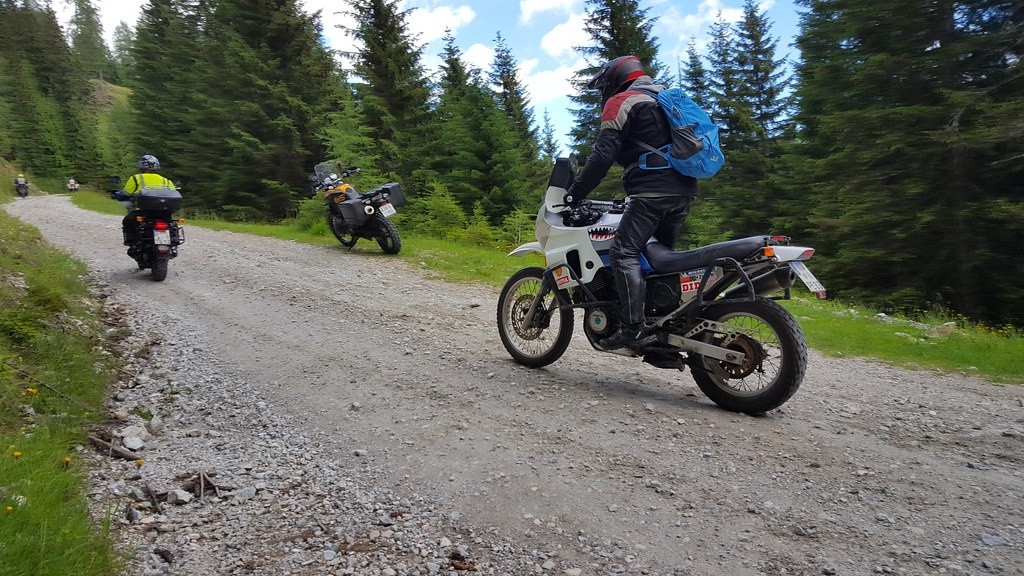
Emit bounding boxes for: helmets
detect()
[587,55,646,112]
[139,154,160,171]
[18,174,24,178]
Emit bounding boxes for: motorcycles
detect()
[111,191,186,282]
[15,180,29,199]
[309,159,408,254]
[496,153,827,414]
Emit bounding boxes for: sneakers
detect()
[600,321,658,352]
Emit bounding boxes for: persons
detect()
[564,55,700,351]
[68,178,77,191]
[114,154,182,256]
[13,173,29,195]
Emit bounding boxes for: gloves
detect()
[563,192,582,210]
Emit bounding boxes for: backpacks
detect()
[628,88,724,179]
